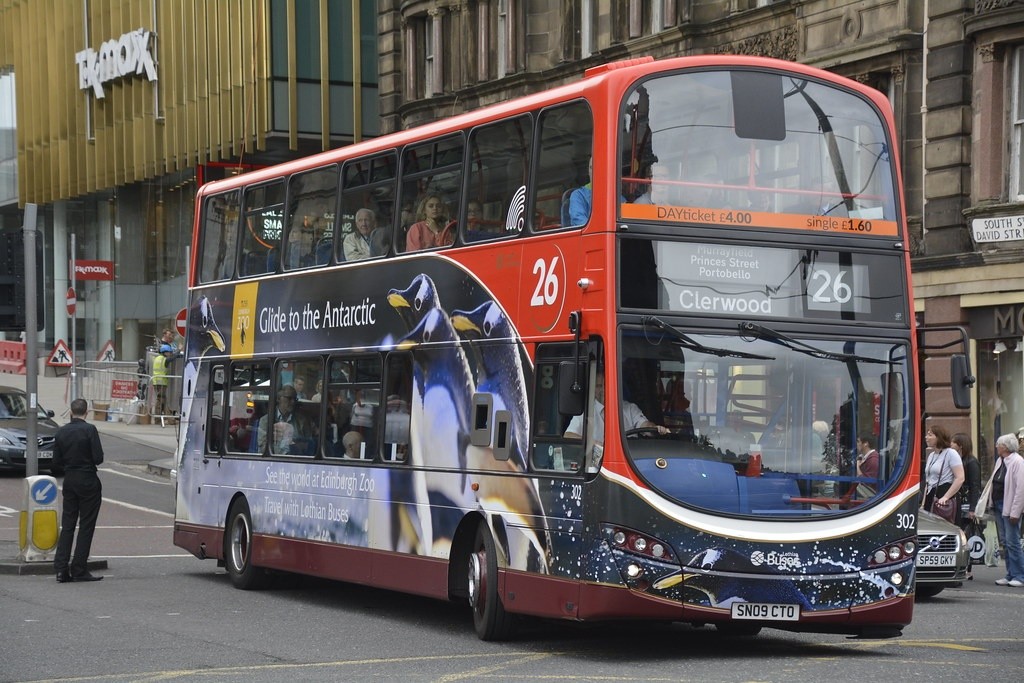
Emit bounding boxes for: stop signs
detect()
[66,288,76,315]
[175,308,187,338]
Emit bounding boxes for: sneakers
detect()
[1007,580,1024,587]
[995,578,1009,586]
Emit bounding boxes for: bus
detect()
[171,54,976,645]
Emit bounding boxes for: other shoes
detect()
[968,574,973,580]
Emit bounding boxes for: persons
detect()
[294,376,308,399]
[51,399,104,583]
[343,201,412,262]
[343,431,367,459]
[951,433,985,565]
[748,176,843,217]
[156,329,175,354]
[405,195,483,252]
[312,380,322,401]
[256,386,319,456]
[569,157,671,226]
[563,364,671,445]
[813,420,879,508]
[152,343,183,425]
[920,425,965,588]
[974,427,1024,586]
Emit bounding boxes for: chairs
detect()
[221,209,561,280]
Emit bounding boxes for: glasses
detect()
[467,207,481,214]
[281,395,298,402]
[646,174,670,179]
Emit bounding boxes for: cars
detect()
[0,386,60,470]
[811,456,972,596]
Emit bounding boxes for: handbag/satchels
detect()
[982,519,1001,567]
[930,495,957,524]
[964,516,985,564]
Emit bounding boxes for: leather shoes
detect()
[72,574,104,581]
[57,571,71,581]
